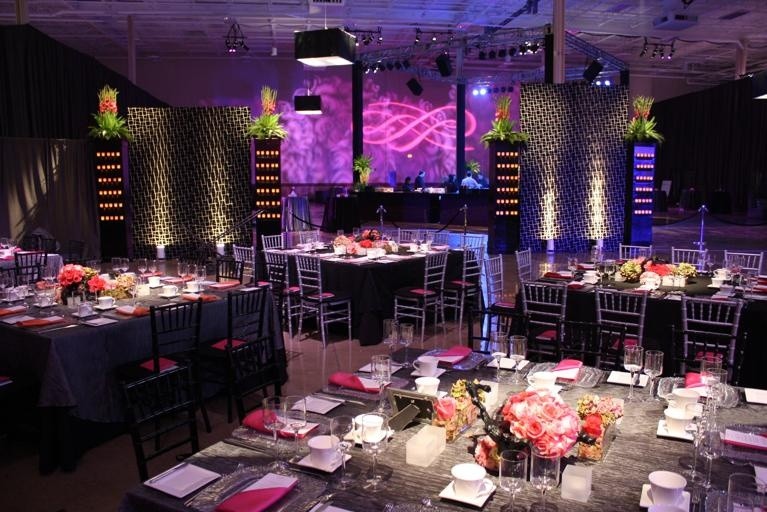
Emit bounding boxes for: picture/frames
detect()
[387,387,438,432]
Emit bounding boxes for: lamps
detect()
[224,23,246,53]
[499,46,506,57]
[651,44,658,58]
[509,45,517,55]
[295,6,355,66]
[489,47,495,59]
[395,60,401,70]
[362,64,369,73]
[379,63,385,71]
[403,58,410,68]
[371,64,378,73]
[640,43,646,56]
[478,47,485,60]
[659,45,664,59]
[294,69,322,115]
[668,46,674,58]
[387,58,393,71]
[530,41,539,53]
[520,42,528,54]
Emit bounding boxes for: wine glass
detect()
[286,396,305,461]
[17,276,29,309]
[148,260,159,277]
[491,332,507,385]
[86,260,101,277]
[644,350,663,403]
[605,260,614,286]
[399,324,412,368]
[361,414,387,492]
[112,257,120,281]
[121,258,129,275]
[740,273,756,302]
[591,246,599,268]
[623,345,643,400]
[137,259,147,285]
[0,271,8,303]
[682,356,727,507]
[262,397,288,470]
[177,263,187,289]
[195,266,206,291]
[509,335,525,384]
[426,235,432,251]
[370,355,390,413]
[383,319,398,355]
[45,286,56,315]
[3,277,14,306]
[187,264,196,282]
[499,450,527,512]
[128,282,136,308]
[568,258,577,278]
[530,445,559,511]
[35,287,45,315]
[330,416,355,489]
[705,254,714,273]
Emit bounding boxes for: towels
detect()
[720,429,766,451]
[243,409,320,439]
[576,263,593,270]
[544,271,575,279]
[432,346,472,365]
[213,473,298,512]
[328,370,392,393]
[165,275,192,284]
[183,293,211,302]
[685,371,715,401]
[140,272,162,277]
[0,305,27,317]
[209,281,240,290]
[568,281,584,288]
[117,305,148,316]
[553,359,583,385]
[632,287,652,296]
[17,316,64,327]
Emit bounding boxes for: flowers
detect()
[675,262,696,281]
[460,381,581,474]
[621,260,640,281]
[334,236,352,247]
[355,229,383,243]
[58,264,104,301]
[360,240,371,248]
[648,264,672,277]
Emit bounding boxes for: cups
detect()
[355,414,383,442]
[162,285,177,295]
[373,240,380,263]
[412,356,439,376]
[148,277,160,286]
[648,471,686,505]
[727,473,765,511]
[336,230,343,237]
[307,435,342,466]
[451,463,492,500]
[414,376,439,395]
[353,228,359,237]
[186,282,200,291]
[418,234,423,244]
[0,238,9,253]
[663,388,698,433]
[77,302,92,327]
[411,233,418,245]
[98,296,115,308]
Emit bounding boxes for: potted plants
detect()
[354,156,376,191]
[624,96,664,146]
[88,85,131,142]
[482,96,527,150]
[246,87,284,139]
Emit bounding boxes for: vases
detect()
[61,289,96,304]
[487,447,563,477]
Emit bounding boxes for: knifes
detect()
[149,462,188,483]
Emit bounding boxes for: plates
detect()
[744,388,766,403]
[80,318,118,326]
[487,356,529,370]
[639,484,690,511]
[656,420,698,441]
[297,454,351,473]
[438,479,496,507]
[144,461,221,498]
[321,372,408,400]
[416,348,493,371]
[290,393,344,414]
[191,465,327,511]
[657,377,737,409]
[718,421,767,463]
[607,370,648,387]
[527,361,602,387]
[182,289,204,292]
[343,429,394,444]
[410,368,445,377]
[157,293,181,298]
[145,283,164,288]
[93,305,118,310]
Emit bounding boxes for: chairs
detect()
[724,249,764,277]
[556,318,627,374]
[15,251,47,282]
[670,246,708,271]
[198,288,269,424]
[299,230,320,249]
[618,242,653,264]
[292,253,353,346]
[514,247,532,296]
[264,251,310,340]
[0,267,15,286]
[682,331,745,390]
[397,227,418,245]
[118,359,203,483]
[434,245,484,330]
[112,300,212,451]
[13,263,41,286]
[224,335,280,427]
[520,279,585,365]
[426,232,448,246]
[215,259,245,287]
[261,234,286,277]
[394,250,447,346]
[680,293,743,386]
[459,235,486,251]
[483,253,521,353]
[232,244,257,287]
[594,288,648,375]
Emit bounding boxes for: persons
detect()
[461,171,480,189]
[403,177,414,192]
[443,174,457,192]
[477,173,487,187]
[415,170,427,191]
